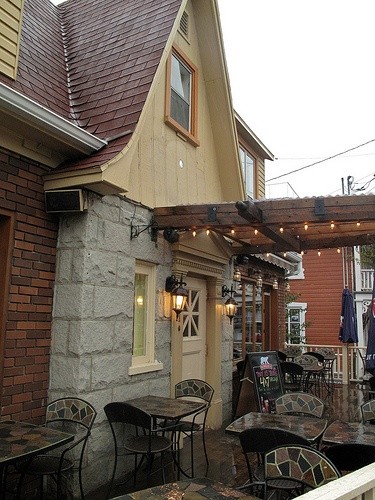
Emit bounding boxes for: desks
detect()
[225,412,328,485]
[322,356,337,393]
[322,420,375,447]
[302,366,322,393]
[0,420,74,500]
[125,395,205,485]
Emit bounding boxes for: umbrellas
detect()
[364,273,375,377]
[339,285,359,389]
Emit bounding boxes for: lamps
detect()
[273,280,278,289]
[234,272,241,281]
[287,283,290,291]
[256,276,263,287]
[222,283,238,325]
[165,272,188,321]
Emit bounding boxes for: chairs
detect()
[16,397,96,499]
[160,380,214,478]
[237,348,375,500]
[103,402,172,498]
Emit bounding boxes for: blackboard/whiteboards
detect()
[234,351,283,413]
